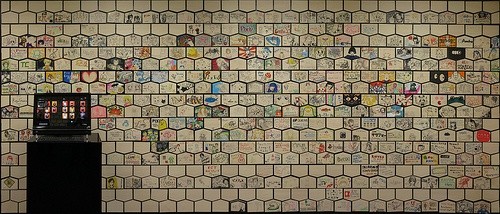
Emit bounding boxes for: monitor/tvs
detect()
[32,93,91,134]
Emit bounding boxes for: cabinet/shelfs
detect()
[25,132,103,212]
